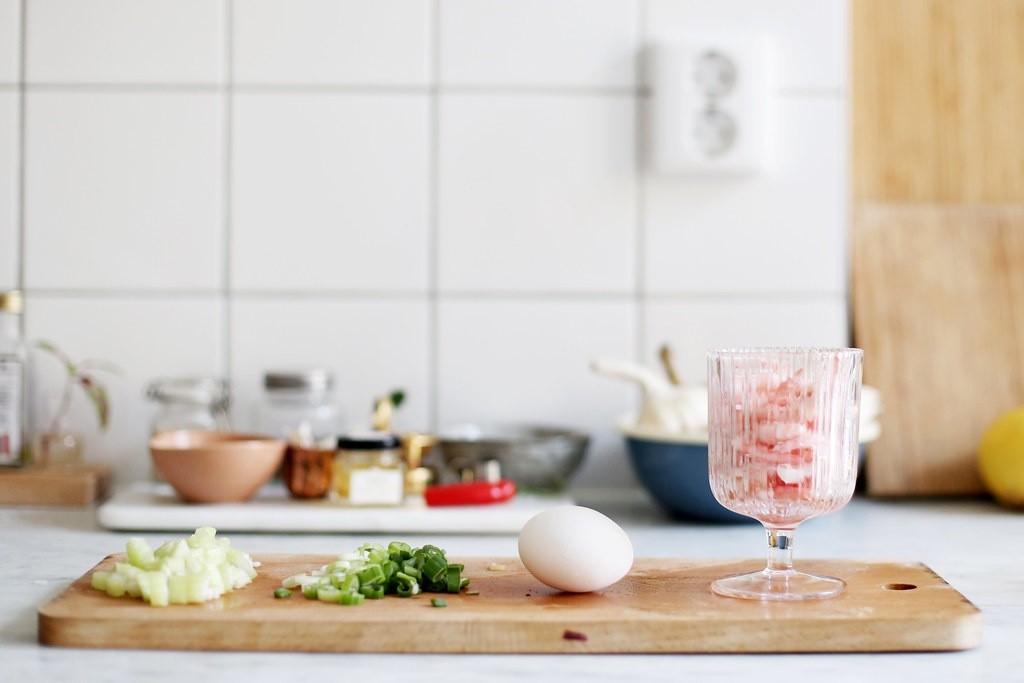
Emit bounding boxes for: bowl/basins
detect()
[618,408,883,528]
[147,430,288,503]
[436,423,595,492]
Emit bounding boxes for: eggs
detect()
[517,507,633,592]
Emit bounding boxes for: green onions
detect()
[275,539,469,606]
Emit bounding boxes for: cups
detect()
[705,347,864,602]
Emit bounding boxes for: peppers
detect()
[426,481,514,506]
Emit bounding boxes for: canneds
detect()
[331,434,403,497]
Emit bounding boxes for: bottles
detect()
[144,377,230,501]
[327,434,406,506]
[0,290,29,468]
[252,365,347,435]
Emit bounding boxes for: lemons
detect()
[979,406,1024,507]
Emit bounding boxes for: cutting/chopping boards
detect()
[37,550,983,654]
